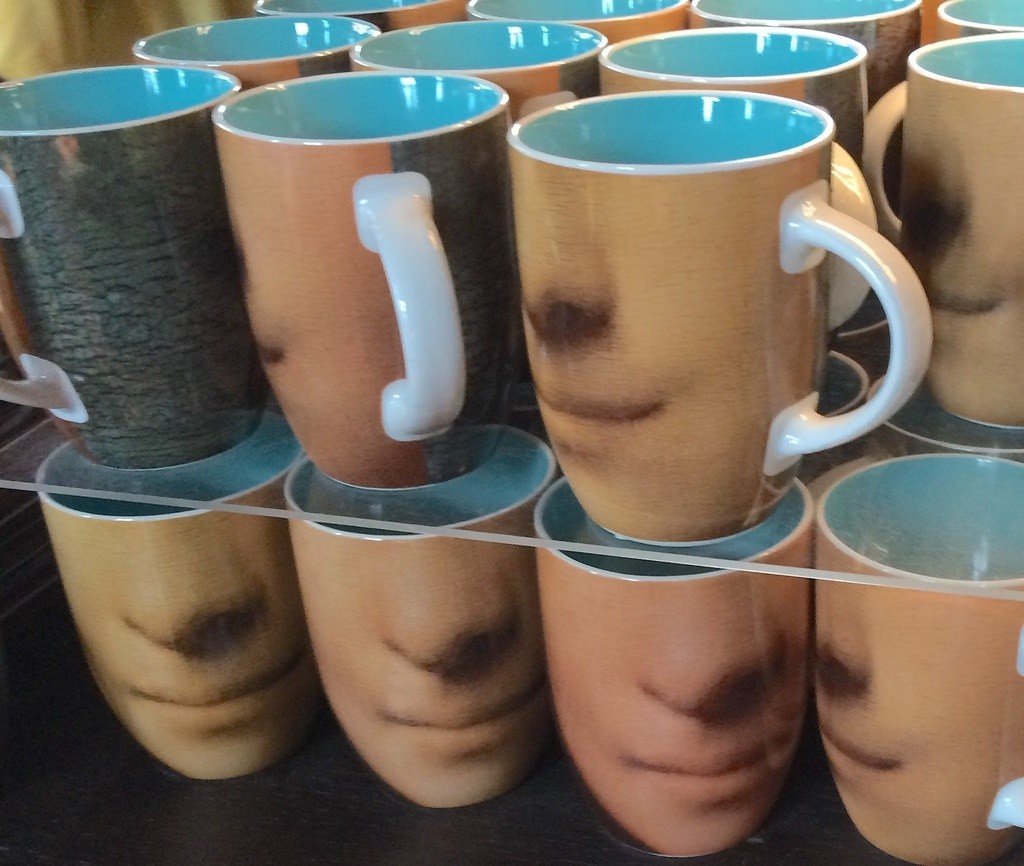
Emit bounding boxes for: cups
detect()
[0,0,1024,548]
[34,300,1024,866]
